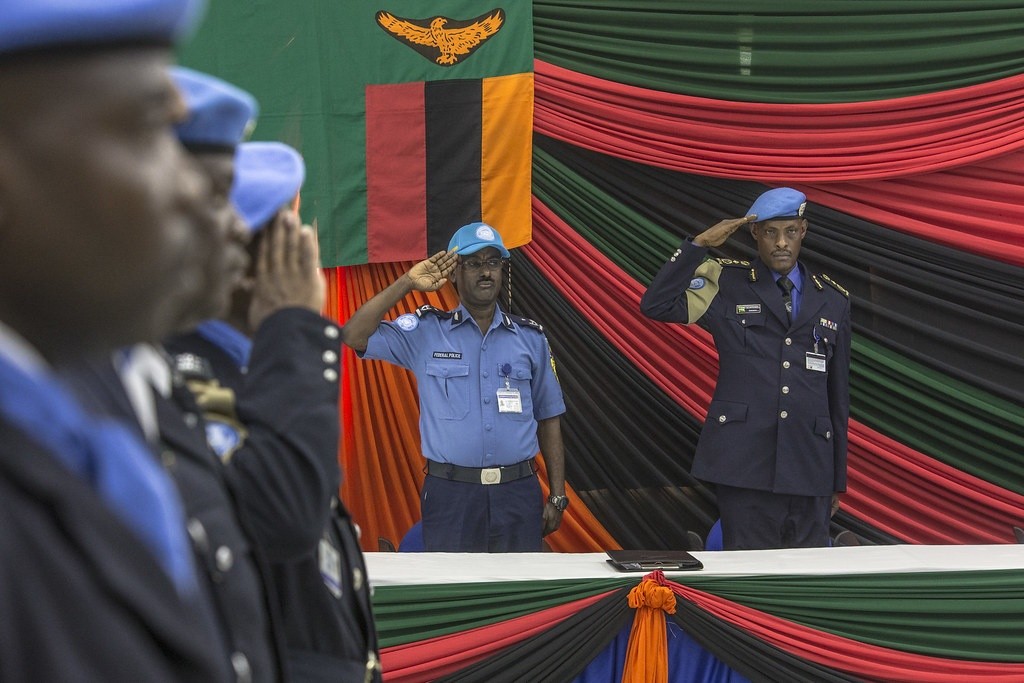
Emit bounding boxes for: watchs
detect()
[548,494,569,511]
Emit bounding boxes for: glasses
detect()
[456,258,507,272]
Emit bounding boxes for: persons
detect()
[639,189,851,551]
[0,0,385,683]
[341,223,566,552]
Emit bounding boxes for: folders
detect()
[606,549,704,572]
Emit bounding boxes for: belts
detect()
[427,458,535,485]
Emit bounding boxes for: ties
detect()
[776,275,795,328]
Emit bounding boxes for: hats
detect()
[447,222,512,259]
[226,141,306,233]
[166,62,262,154]
[0,0,209,66]
[743,186,807,222]
